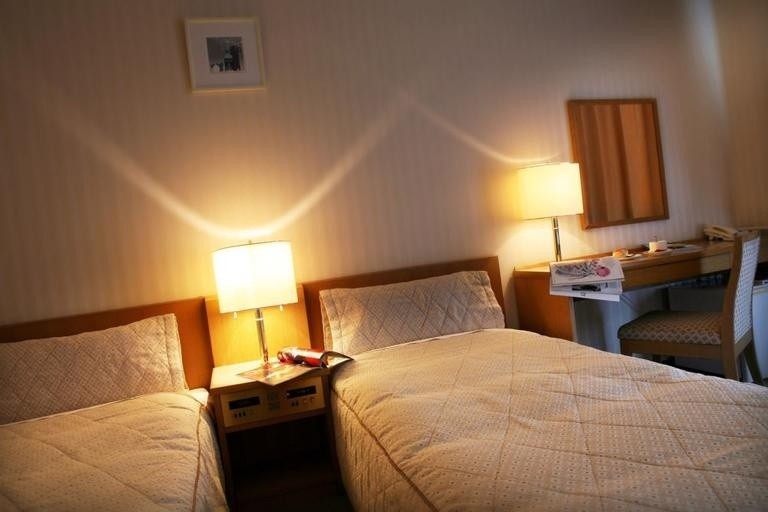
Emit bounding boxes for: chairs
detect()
[616,228,765,385]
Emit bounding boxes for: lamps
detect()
[212,240,299,366]
[516,162,585,262]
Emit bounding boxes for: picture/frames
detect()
[183,15,266,94]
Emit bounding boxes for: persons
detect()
[222,41,241,70]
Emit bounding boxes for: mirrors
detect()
[567,97,671,231]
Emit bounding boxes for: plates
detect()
[602,252,641,261]
[643,248,673,256]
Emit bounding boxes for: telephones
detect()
[704,225,740,241]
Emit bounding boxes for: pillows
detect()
[318,271,505,365]
[0,313,189,424]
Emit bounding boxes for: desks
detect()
[513,228,767,376]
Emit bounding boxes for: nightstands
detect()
[208,364,338,494]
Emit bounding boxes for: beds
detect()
[302,256,768,511]
[0,296,230,511]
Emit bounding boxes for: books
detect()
[549,255,626,303]
[237,346,354,388]
